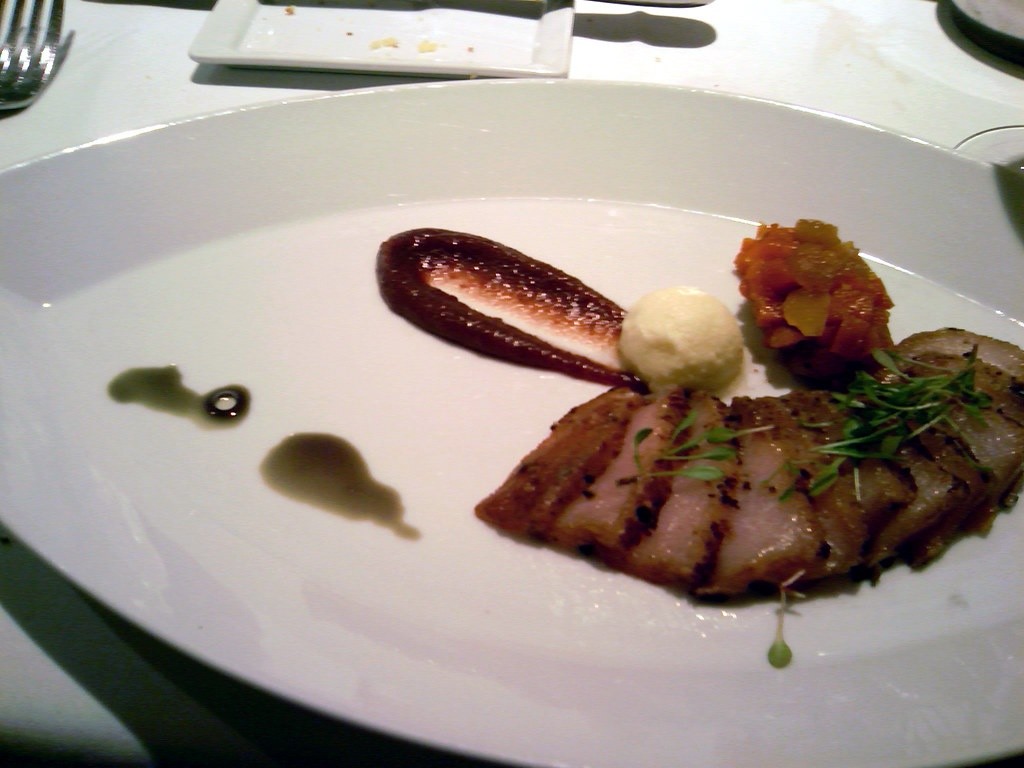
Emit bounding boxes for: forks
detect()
[0,0,65,113]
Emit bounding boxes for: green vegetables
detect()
[632,345,994,666]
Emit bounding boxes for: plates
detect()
[0,77,1024,766]
[188,0,576,80]
[952,126,1024,177]
